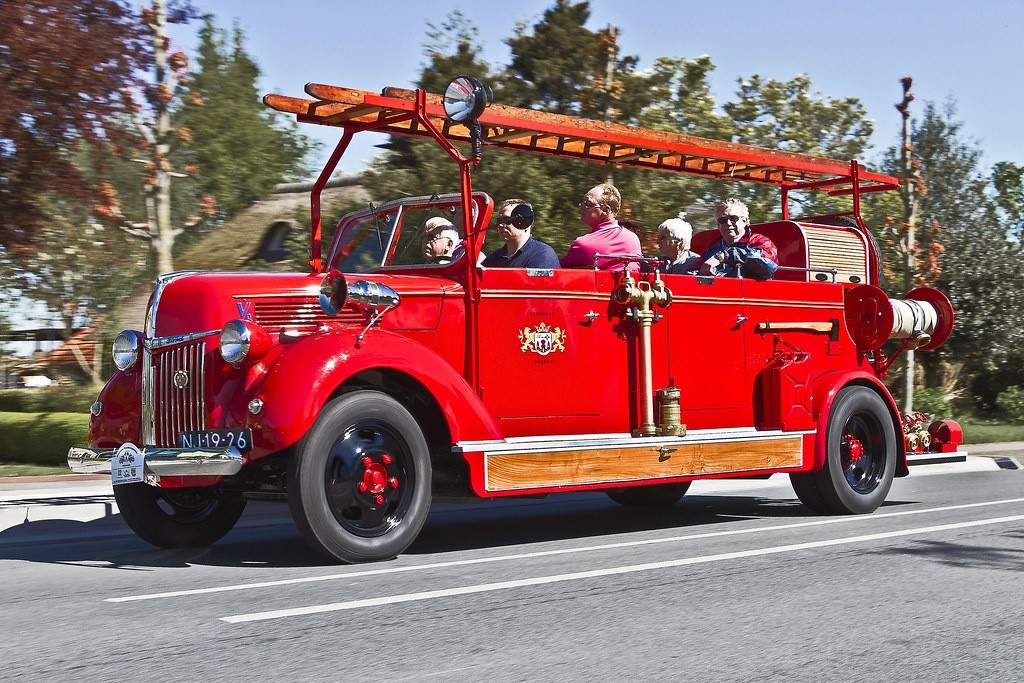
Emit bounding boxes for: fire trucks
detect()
[66,77,956,564]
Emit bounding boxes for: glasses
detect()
[717,215,743,223]
[581,199,604,208]
[421,234,445,241]
[497,216,512,225]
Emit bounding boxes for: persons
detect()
[618,222,642,245]
[421,217,460,265]
[679,197,779,280]
[560,184,644,271]
[640,218,701,275]
[481,198,561,270]
